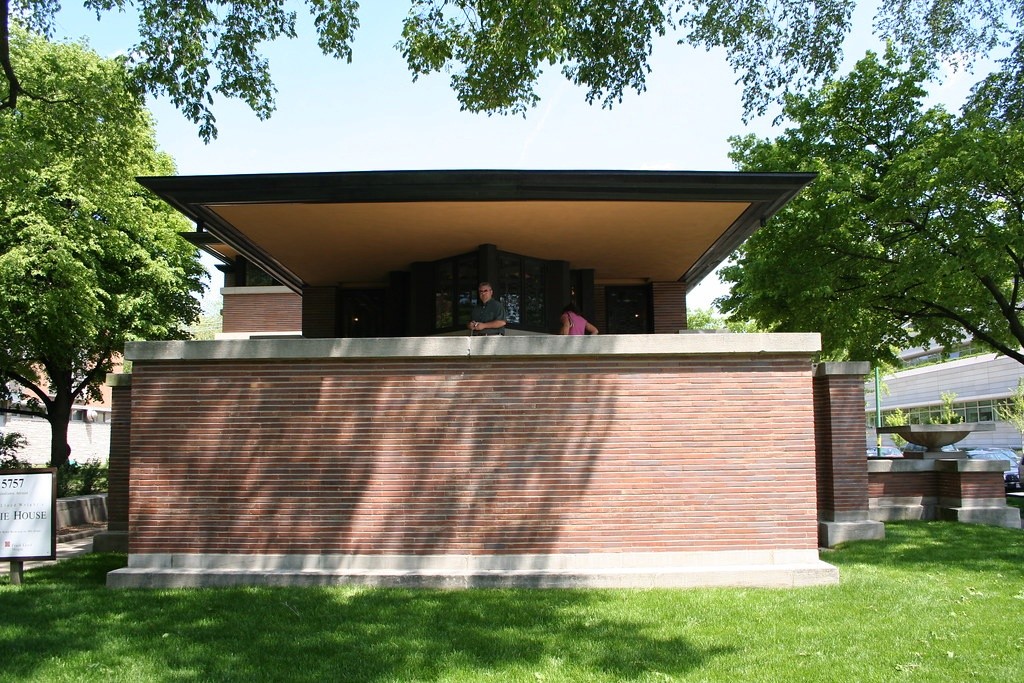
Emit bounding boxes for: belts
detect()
[478,333,503,336]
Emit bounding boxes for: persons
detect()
[560,302,599,335]
[467,282,507,337]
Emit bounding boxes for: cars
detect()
[866,441,1024,494]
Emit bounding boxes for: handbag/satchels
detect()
[560,312,574,335]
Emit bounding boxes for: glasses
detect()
[479,289,492,293]
